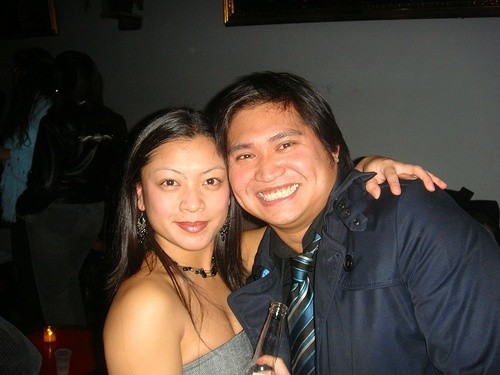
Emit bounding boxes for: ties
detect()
[287,232,320,375]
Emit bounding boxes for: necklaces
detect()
[172,256,217,277]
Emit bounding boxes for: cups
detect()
[55,349,71,375]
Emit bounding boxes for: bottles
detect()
[242,301,289,375]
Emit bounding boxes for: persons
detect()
[15,51,129,328]
[79,186,118,321]
[102,107,447,375]
[0,45,60,287]
[206,68,500,375]
